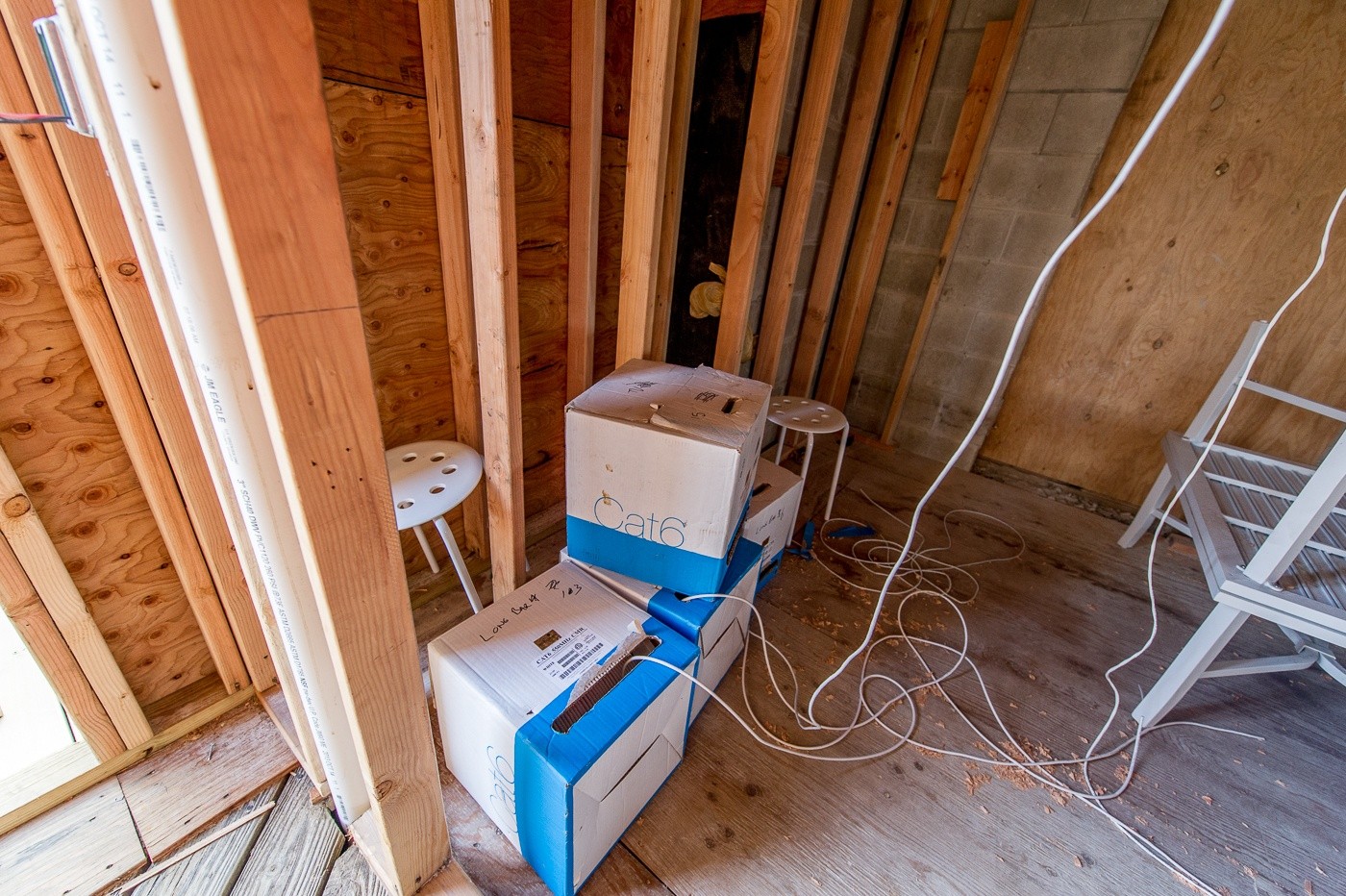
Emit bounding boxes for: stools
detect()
[769,394,849,520]
[385,440,483,615]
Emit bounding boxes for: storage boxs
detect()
[426,359,803,896]
